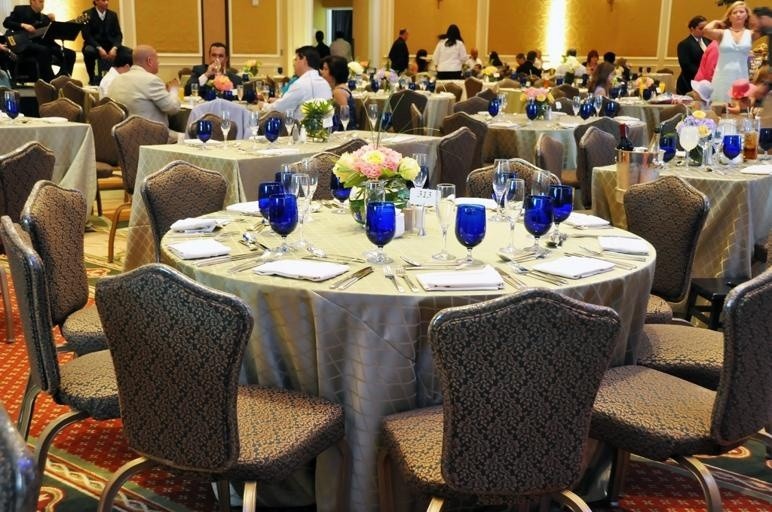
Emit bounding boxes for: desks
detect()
[161,185,657,512]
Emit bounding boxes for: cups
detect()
[214,58,222,71]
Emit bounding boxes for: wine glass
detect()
[4,90,20,126]
[259,183,283,248]
[523,195,553,256]
[268,193,299,258]
[411,153,430,165]
[193,103,392,149]
[280,164,300,198]
[342,76,437,98]
[455,205,487,269]
[487,65,671,124]
[531,170,550,203]
[330,168,352,215]
[275,171,298,192]
[547,185,573,241]
[658,112,772,175]
[178,73,276,106]
[287,173,318,247]
[491,170,517,212]
[432,184,457,260]
[406,166,431,189]
[500,179,525,253]
[488,159,512,223]
[366,202,396,266]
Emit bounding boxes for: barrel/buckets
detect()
[613,146,665,204]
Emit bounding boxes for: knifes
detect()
[196,247,648,294]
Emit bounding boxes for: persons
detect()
[184,42,242,101]
[82,0,123,85]
[0,0,76,86]
[107,46,186,144]
[98,46,133,100]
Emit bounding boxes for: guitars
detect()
[1,12,91,71]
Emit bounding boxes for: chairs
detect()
[592,265,772,511]
[0,214,122,512]
[0,53,772,345]
[95,262,346,512]
[381,286,623,511]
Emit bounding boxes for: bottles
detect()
[644,126,661,153]
[617,122,633,150]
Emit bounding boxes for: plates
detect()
[39,116,68,124]
